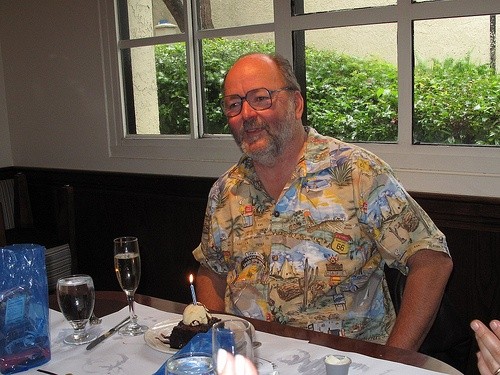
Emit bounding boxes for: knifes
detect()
[86,315,138,350]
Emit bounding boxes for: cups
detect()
[323,355,351,375]
[165,352,216,375]
[212,318,255,375]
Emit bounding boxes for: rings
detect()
[494,369,500,375]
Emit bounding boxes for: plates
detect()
[144,314,255,353]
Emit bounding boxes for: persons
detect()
[192,51,453,351]
[470,320,500,375]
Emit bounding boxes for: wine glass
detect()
[114,236,148,337]
[56,274,98,345]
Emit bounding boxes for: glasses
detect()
[218,86,296,118]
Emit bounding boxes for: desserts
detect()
[169,302,224,348]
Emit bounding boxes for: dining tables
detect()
[12,289,464,375]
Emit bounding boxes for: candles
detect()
[189,274,197,304]
